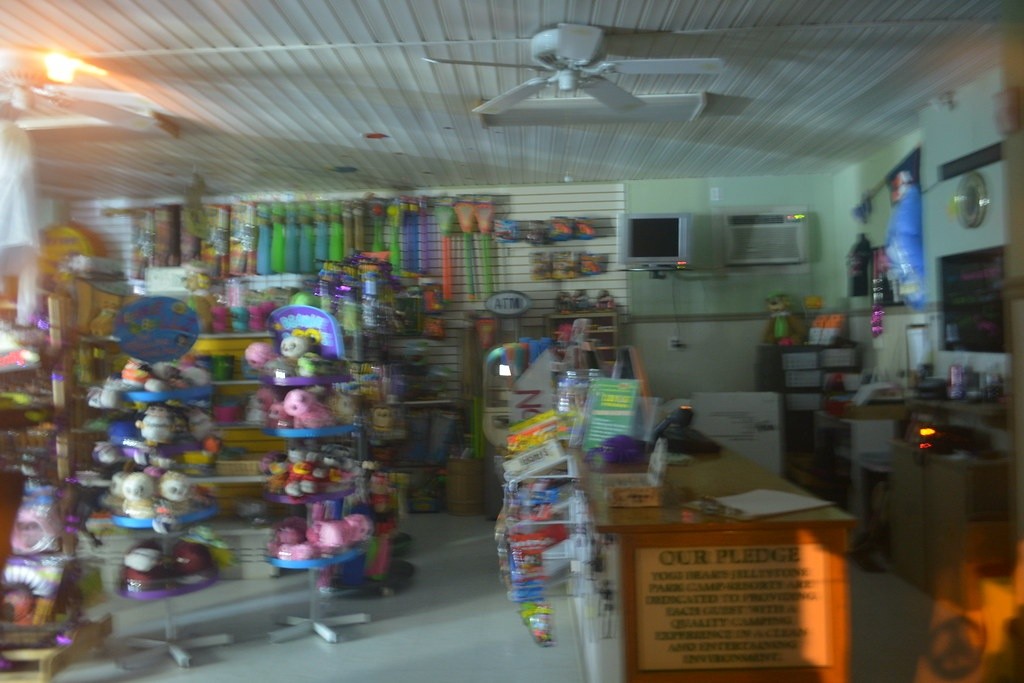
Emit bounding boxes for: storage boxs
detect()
[781,348,863,413]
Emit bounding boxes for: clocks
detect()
[955,172,988,229]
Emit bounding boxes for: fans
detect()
[419,22,721,115]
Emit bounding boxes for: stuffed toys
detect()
[243,333,373,561]
[88,359,221,600]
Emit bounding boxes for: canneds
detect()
[948,365,963,400]
